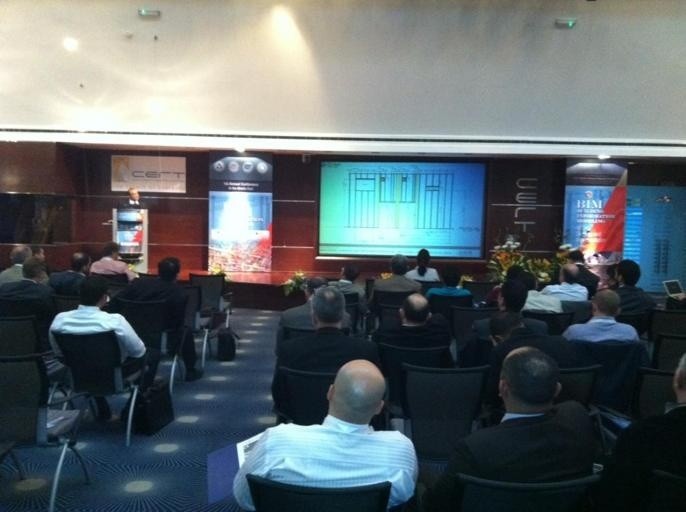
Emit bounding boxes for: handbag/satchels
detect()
[135,379,174,435]
[217,327,235,361]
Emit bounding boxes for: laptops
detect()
[662,280,686,301]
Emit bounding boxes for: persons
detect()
[108,187,145,224]
[1,239,205,424]
[229,241,684,511]
